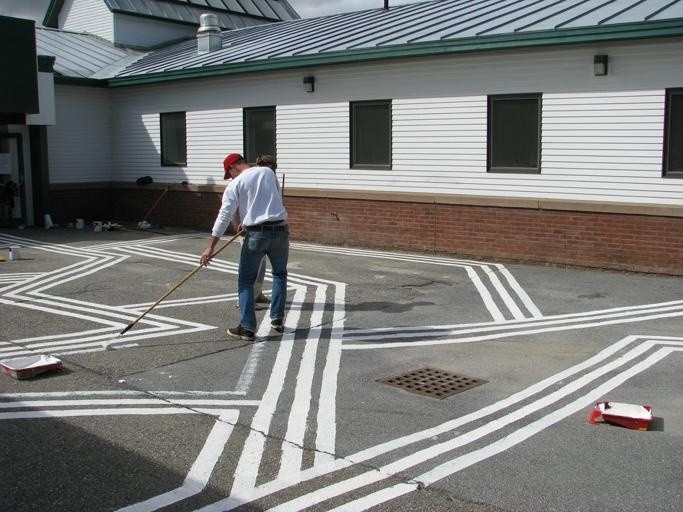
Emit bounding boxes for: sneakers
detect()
[227,324,254,341]
[257,294,269,302]
[237,301,262,311]
[270,318,282,330]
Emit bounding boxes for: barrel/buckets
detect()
[92,220,104,232]
[73,219,85,228]
[8,246,21,259]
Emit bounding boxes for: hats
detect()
[223,153,240,179]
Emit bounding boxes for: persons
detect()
[197,153,290,340]
[228,154,277,311]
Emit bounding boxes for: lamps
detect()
[303,76,315,93]
[594,55,608,76]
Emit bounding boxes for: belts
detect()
[246,224,289,231]
